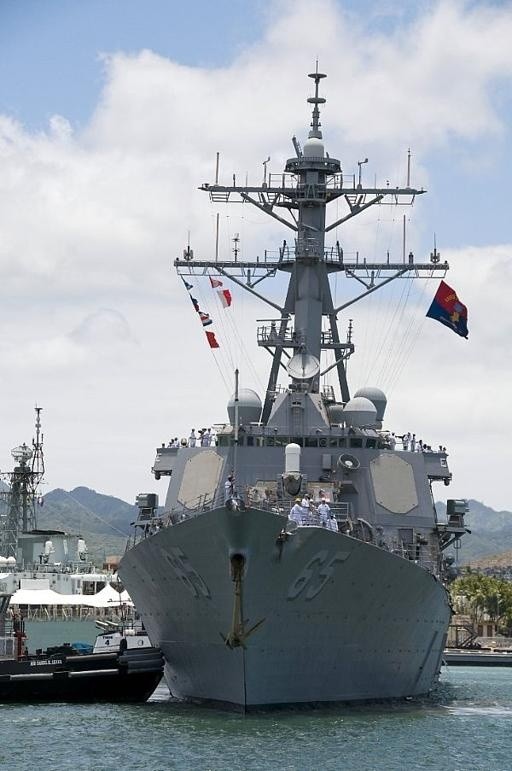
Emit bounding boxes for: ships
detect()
[116,59,469,710]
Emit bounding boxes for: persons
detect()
[388,430,445,454]
[161,427,216,447]
[147,475,339,538]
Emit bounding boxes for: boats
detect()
[0,402,165,702]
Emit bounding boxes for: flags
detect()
[209,277,223,289]
[215,289,232,308]
[425,280,470,340]
[190,294,200,313]
[182,277,193,290]
[198,311,213,327]
[205,331,220,349]
